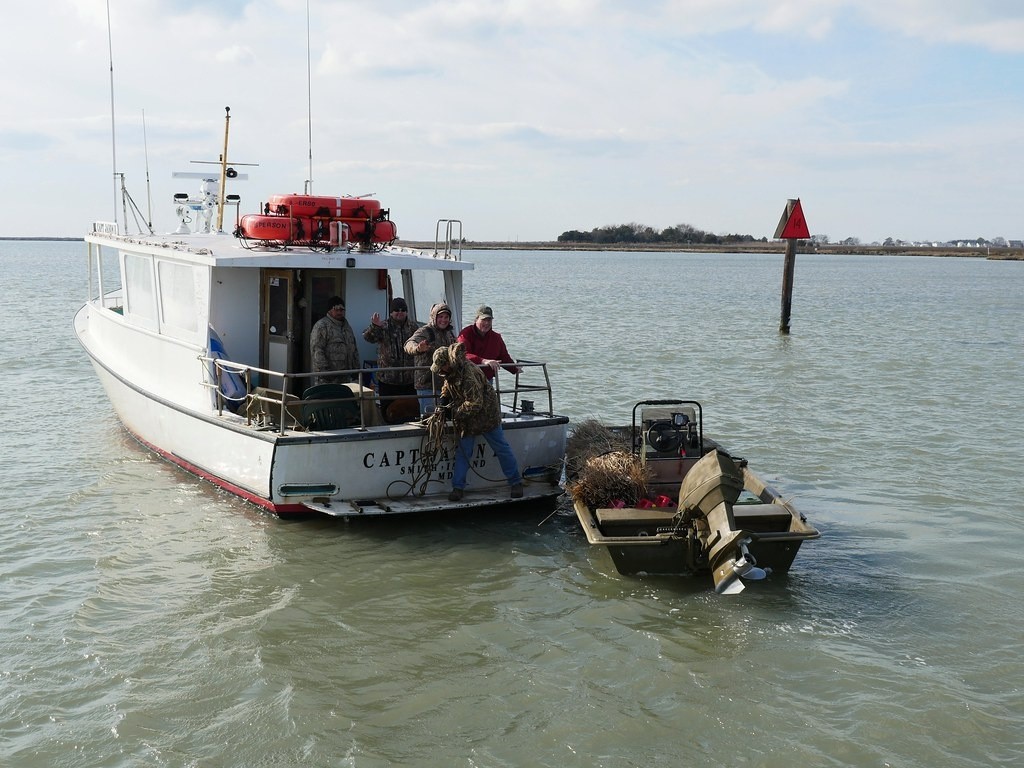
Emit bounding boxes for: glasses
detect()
[333,305,345,310]
[394,307,406,312]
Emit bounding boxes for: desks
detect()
[340,383,382,427]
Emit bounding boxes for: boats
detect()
[71,0,571,519]
[564,397,821,597]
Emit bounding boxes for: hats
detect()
[329,298,345,311]
[430,346,449,374]
[437,310,450,315]
[475,304,493,320]
[390,298,407,313]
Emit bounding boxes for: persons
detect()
[430,346,523,501]
[309,296,360,386]
[363,297,524,422]
[269,279,308,395]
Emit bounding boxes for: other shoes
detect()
[449,487,463,501]
[511,482,523,497]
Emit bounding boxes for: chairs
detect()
[237,384,361,432]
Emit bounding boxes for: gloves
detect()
[436,396,452,421]
